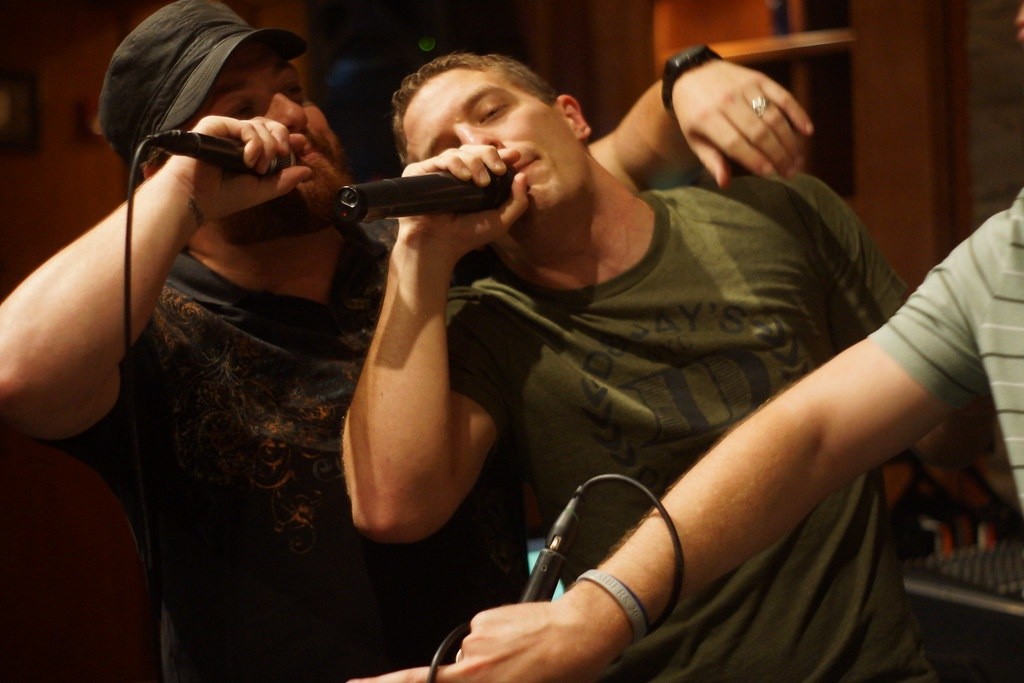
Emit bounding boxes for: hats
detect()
[99,0,306,166]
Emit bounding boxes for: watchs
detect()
[662,44,724,120]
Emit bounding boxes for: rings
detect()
[752,96,769,117]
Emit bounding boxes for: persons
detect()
[0,0,915,683]
[348,189,1024,683]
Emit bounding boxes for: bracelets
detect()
[576,569,650,643]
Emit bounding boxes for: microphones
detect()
[331,163,521,226]
[145,129,298,178]
[522,493,589,602]
